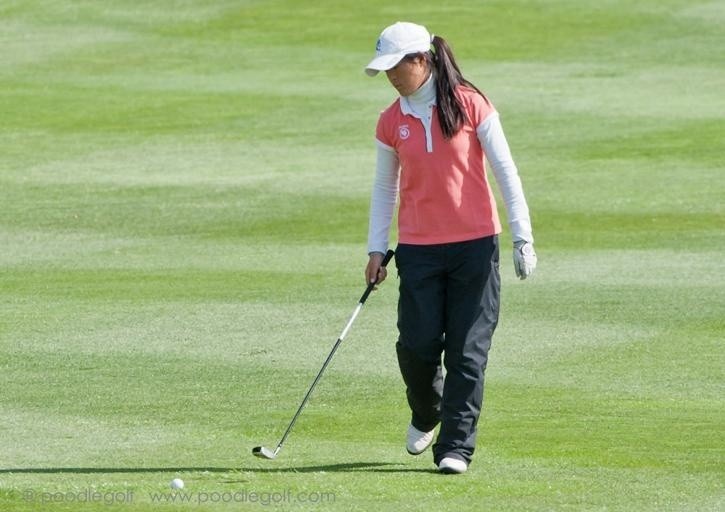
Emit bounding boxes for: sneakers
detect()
[439,456,467,474]
[406,422,434,454]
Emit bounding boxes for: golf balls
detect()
[172,479,183,490]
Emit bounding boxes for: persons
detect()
[362,19,540,473]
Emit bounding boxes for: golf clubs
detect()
[252,249,395,459]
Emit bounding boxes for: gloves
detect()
[512,240,538,279]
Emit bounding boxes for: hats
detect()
[365,22,431,78]
